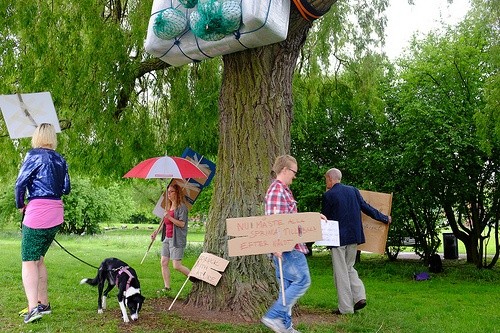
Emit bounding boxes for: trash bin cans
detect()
[442,232,458,260]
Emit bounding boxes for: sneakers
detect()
[260,316,288,333]
[157,287,171,293]
[189,276,202,284]
[19,301,52,317]
[24,306,43,324]
[288,323,299,333]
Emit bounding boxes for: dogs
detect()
[79,257,146,323]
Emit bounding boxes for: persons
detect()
[15,122,71,324]
[150,183,191,292]
[320,168,391,315]
[259,156,328,333]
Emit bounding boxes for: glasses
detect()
[282,168,299,176]
[167,191,176,193]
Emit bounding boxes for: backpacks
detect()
[427,254,444,273]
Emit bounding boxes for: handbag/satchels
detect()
[412,271,431,281]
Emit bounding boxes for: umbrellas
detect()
[121,151,209,213]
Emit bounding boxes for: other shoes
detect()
[354,300,367,310]
[331,309,346,315]
